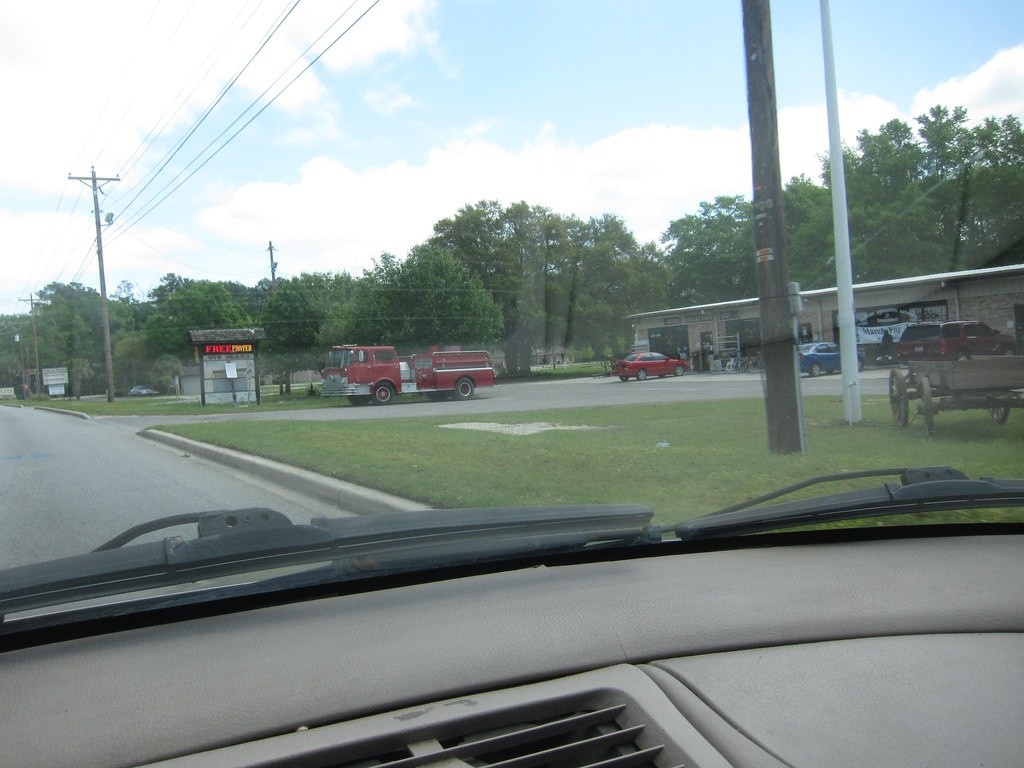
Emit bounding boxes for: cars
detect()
[798,342,866,377]
[130,385,152,397]
[610,352,687,381]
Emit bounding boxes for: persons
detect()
[882,331,894,352]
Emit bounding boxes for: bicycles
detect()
[728,348,763,373]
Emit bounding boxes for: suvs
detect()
[896,320,1017,367]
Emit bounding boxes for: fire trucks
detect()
[320,345,497,406]
